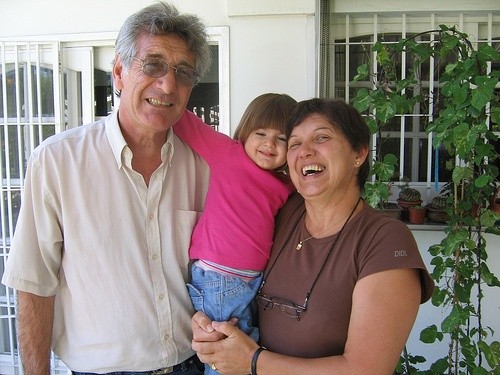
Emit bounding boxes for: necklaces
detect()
[296,207,347,249]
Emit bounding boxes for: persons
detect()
[1,1,211,375]
[189,96,433,375]
[174,93,299,375]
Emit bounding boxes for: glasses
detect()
[132,54,200,88]
[256,282,309,321]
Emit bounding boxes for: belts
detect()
[146,355,198,375]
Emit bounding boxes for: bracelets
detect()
[251,346,268,375]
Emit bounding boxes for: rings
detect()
[212,363,217,370]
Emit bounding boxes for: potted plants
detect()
[379,180,500,225]
